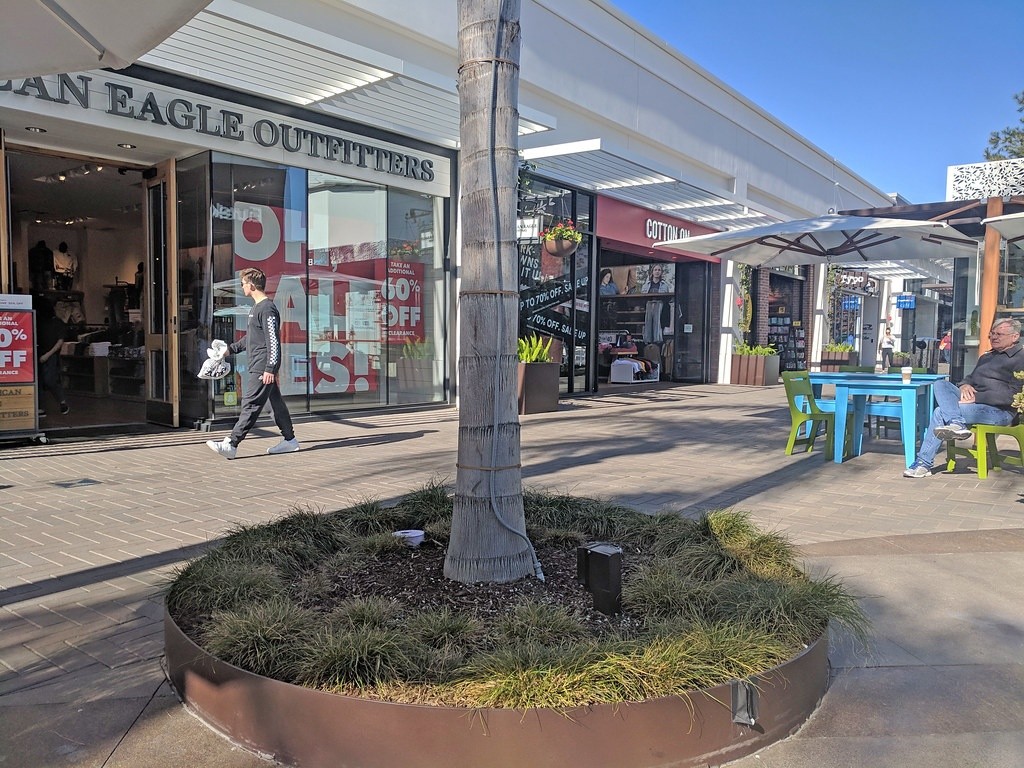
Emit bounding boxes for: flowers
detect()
[538,220,582,244]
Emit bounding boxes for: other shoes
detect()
[61,403,69,414]
[38,412,46,418]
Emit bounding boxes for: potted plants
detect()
[891,351,910,367]
[820,351,858,375]
[517,332,560,415]
[731,344,780,385]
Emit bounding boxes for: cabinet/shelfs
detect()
[60,352,146,402]
[603,292,676,342]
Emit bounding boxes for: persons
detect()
[903,319,1024,478]
[944,331,951,363]
[29,240,78,290]
[642,265,669,294]
[879,328,895,372]
[206,267,300,459]
[35,301,69,418]
[600,269,619,295]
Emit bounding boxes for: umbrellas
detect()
[653,209,979,436]
[0,0,215,80]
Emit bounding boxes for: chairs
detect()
[946,414,1024,479]
[780,370,854,461]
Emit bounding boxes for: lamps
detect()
[35,163,276,225]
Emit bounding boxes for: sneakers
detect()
[206,437,237,460]
[933,420,972,441]
[267,436,300,454]
[903,460,932,478]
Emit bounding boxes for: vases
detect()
[544,240,578,258]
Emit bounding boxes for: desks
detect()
[794,372,949,470]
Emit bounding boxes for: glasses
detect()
[240,282,254,287]
[990,331,1014,338]
